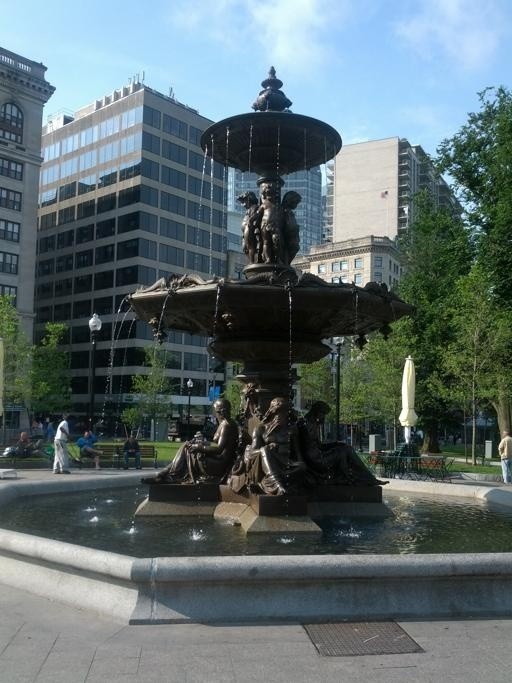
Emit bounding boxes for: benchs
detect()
[80,446,158,469]
[1,446,54,469]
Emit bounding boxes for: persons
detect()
[138,397,239,484]
[16,431,43,455]
[236,188,262,263]
[253,184,283,263]
[123,435,141,470]
[52,414,74,474]
[76,429,104,469]
[45,416,55,441]
[281,191,302,265]
[249,398,308,497]
[497,429,511,486]
[297,400,390,486]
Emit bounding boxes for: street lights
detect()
[187,379,193,441]
[332,336,344,440]
[88,313,102,431]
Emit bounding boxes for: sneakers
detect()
[94,449,104,470]
[123,464,142,471]
[52,468,73,475]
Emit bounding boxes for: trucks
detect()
[168,416,214,442]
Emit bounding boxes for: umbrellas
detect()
[398,354,418,442]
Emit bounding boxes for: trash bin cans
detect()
[369,434,381,453]
[485,440,497,458]
